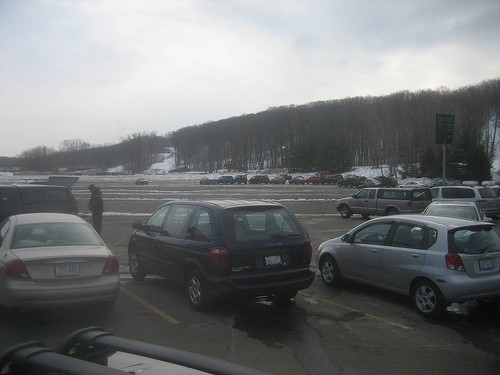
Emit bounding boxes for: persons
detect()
[88,184,104,235]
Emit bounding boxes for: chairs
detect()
[393,225,413,248]
[60,224,78,242]
[412,228,428,246]
[18,226,34,241]
[468,232,486,251]
[232,212,250,236]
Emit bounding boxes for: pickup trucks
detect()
[335,184,432,218]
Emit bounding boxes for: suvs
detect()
[0,175,80,225]
[128,199,316,313]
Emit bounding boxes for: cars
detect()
[0,213,121,320]
[135,178,148,185]
[314,213,499,319]
[199,172,429,189]
[416,186,500,217]
[410,200,493,243]
